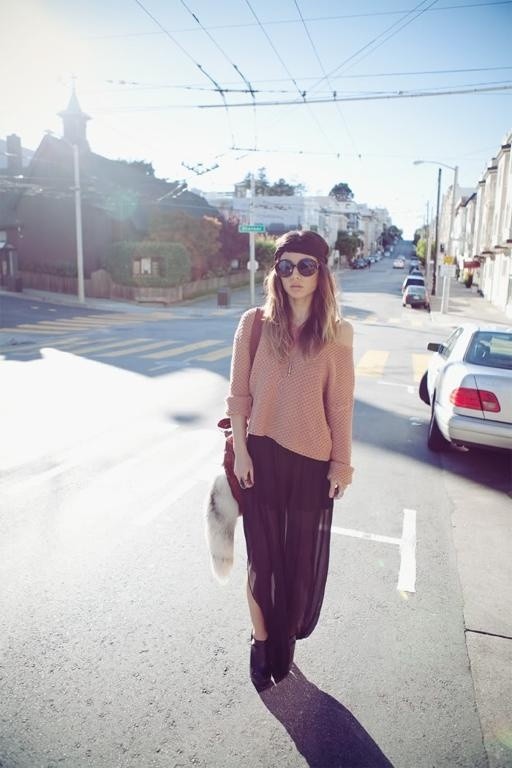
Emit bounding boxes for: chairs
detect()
[475,343,490,363]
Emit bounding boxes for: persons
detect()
[214,228,360,696]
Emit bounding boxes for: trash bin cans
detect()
[217,287,232,309]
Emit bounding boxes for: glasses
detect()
[275,257,323,278]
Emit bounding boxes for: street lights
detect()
[412,159,459,313]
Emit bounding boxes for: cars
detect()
[351,254,382,269]
[419,321,511,451]
[393,255,427,308]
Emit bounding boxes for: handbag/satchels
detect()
[223,432,250,520]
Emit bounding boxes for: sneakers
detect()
[249,632,272,687]
